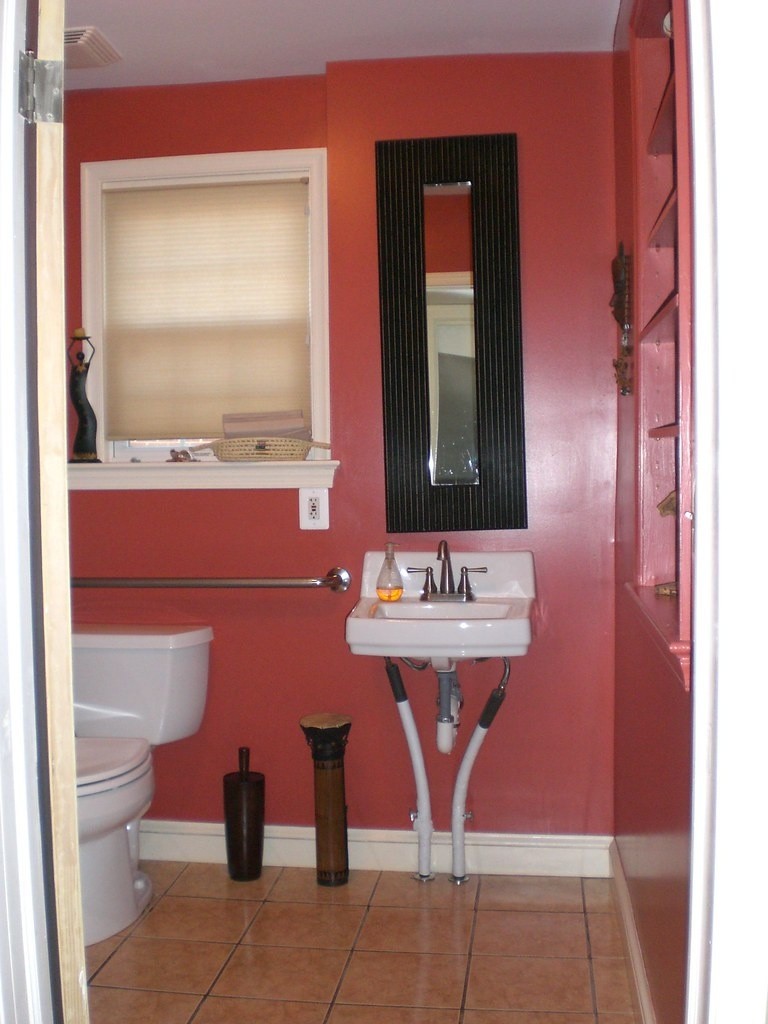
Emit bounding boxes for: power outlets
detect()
[299,488,329,530]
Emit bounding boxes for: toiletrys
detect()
[376,541,404,603]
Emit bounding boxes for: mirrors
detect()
[370,133,530,533]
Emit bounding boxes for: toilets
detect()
[71,624,214,948]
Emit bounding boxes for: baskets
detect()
[210,437,312,462]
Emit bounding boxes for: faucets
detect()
[437,540,456,595]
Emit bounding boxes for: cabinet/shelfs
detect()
[615,1,692,688]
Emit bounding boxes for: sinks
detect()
[345,550,536,658]
